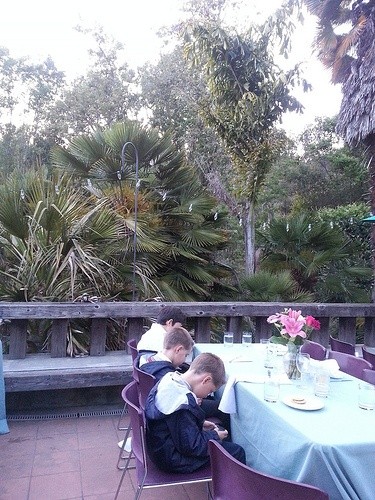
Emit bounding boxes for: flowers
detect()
[267,308,321,346]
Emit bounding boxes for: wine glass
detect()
[295,353,310,389]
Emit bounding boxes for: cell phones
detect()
[211,426,226,431]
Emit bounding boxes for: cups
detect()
[264,364,281,403]
[242,331,252,350]
[314,369,329,398]
[223,331,234,351]
[358,379,375,411]
[259,339,270,351]
[264,342,277,364]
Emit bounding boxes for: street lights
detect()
[116,141,142,301]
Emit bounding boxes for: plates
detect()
[282,393,325,410]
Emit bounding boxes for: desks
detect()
[193,342,375,500]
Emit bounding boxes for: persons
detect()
[144,353,247,475]
[137,306,193,367]
[137,327,232,441]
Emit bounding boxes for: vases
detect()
[283,343,305,380]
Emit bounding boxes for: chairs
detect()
[115,338,375,500]
